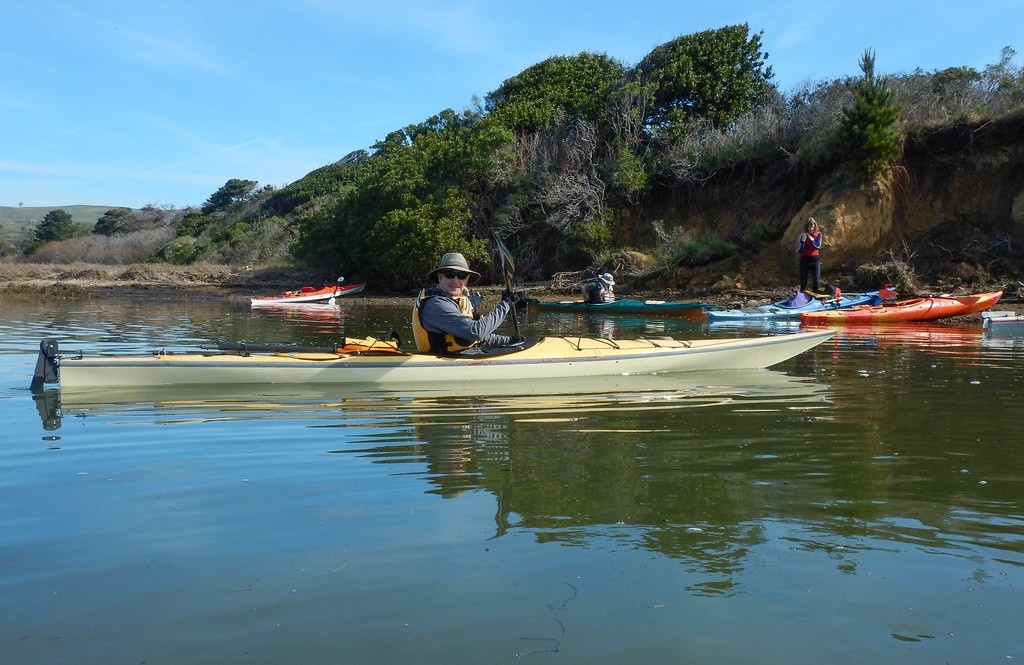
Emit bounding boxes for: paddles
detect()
[803,287,898,300]
[200,343,406,357]
[327,276,345,305]
[918,293,981,306]
[494,234,522,342]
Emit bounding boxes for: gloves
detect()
[510,335,526,344]
[502,290,519,304]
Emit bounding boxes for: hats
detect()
[598,273,615,285]
[429,253,481,284]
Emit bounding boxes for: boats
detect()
[704,283,897,330]
[502,288,719,313]
[250,278,368,309]
[30,327,837,412]
[799,291,1004,326]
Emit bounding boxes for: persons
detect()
[410,252,527,357]
[797,217,822,293]
[582,273,616,302]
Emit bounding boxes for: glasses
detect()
[439,270,471,279]
